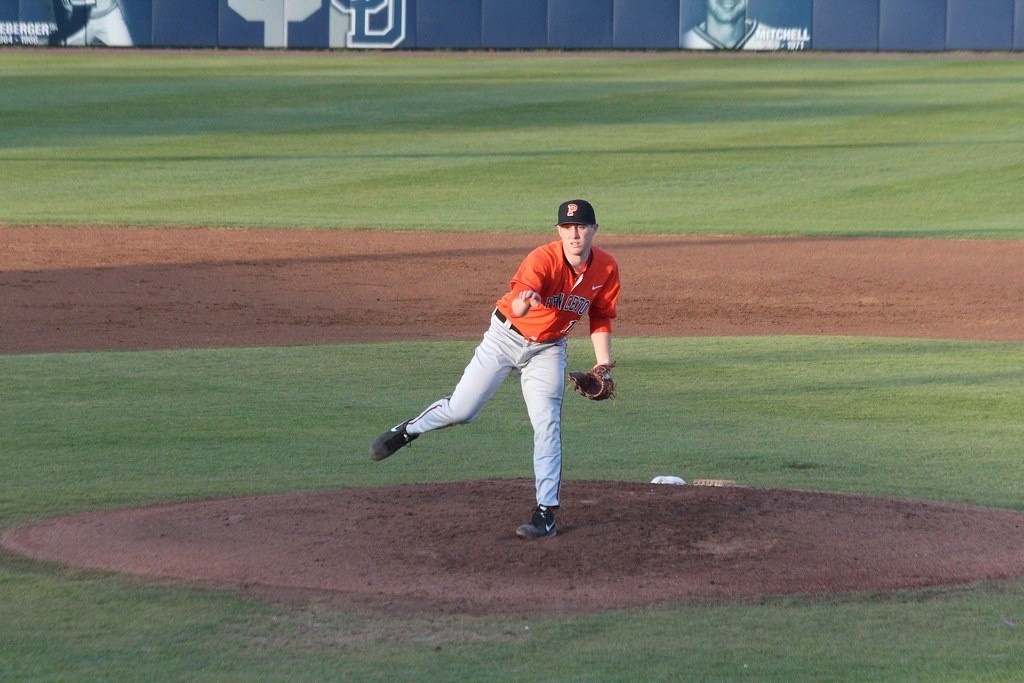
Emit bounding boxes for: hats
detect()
[553,198,596,225]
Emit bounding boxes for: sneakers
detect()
[371,418,419,461]
[515,504,557,539]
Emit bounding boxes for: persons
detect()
[683,0,782,51]
[48,0,134,48]
[370,198,621,539]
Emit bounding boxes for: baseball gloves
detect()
[568,364,615,401]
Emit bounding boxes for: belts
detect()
[495,309,524,337]
